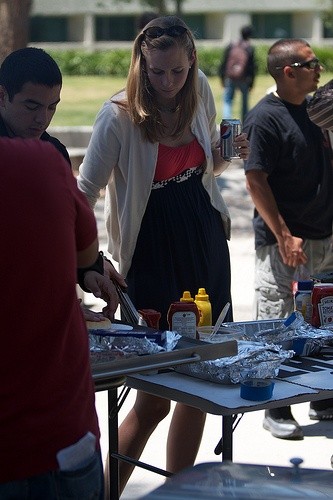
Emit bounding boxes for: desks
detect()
[108,345,333,500]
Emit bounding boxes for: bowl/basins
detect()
[196,325,243,342]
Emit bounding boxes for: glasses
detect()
[143,24,188,38]
[274,58,319,70]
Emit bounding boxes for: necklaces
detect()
[156,103,180,113]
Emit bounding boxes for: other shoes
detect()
[308,399,333,420]
[262,406,303,439]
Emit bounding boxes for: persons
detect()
[241,38,333,439]
[0,47,119,500]
[218,25,259,125]
[306,79,333,130]
[78,16,252,500]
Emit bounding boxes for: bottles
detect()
[194,288,213,334]
[294,280,333,329]
[167,291,200,340]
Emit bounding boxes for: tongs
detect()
[106,259,149,328]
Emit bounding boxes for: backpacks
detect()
[224,41,250,78]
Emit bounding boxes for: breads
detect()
[85,318,111,329]
